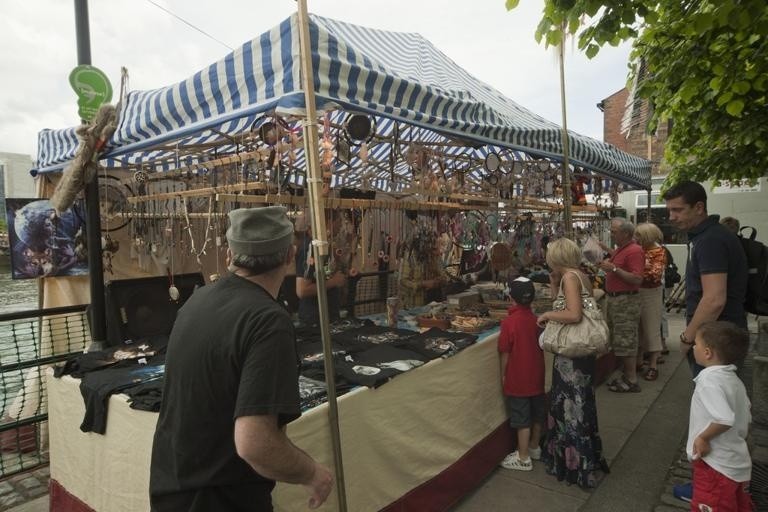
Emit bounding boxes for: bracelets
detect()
[678,332,695,346]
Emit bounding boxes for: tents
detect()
[33,12,653,218]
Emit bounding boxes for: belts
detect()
[608,290,638,297]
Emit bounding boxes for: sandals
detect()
[606,375,626,386]
[608,380,640,394]
[644,367,657,381]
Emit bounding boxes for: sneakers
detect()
[673,483,691,501]
[526,445,541,461]
[497,451,533,471]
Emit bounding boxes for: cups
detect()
[386,296,401,328]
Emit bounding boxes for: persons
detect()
[294,207,348,324]
[497,273,549,473]
[661,180,749,506]
[397,232,440,310]
[12,206,78,278]
[683,319,755,512]
[583,213,675,394]
[536,236,612,492]
[425,234,453,304]
[719,215,740,236]
[148,204,335,511]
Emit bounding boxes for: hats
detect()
[509,276,534,300]
[226,205,296,257]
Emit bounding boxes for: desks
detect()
[35,271,623,512]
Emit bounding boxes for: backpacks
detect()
[738,226,768,316]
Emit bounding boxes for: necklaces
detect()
[99,155,248,303]
[334,198,436,276]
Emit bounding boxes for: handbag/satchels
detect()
[542,292,611,361]
